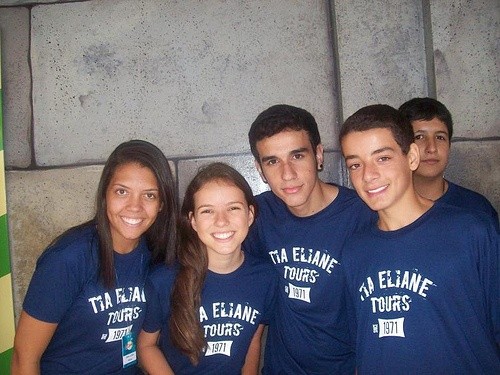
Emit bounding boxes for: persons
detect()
[11,140,182,375]
[138,161,279,375]
[339,104,500,375]
[399,98,500,230]
[244,105,380,375]
